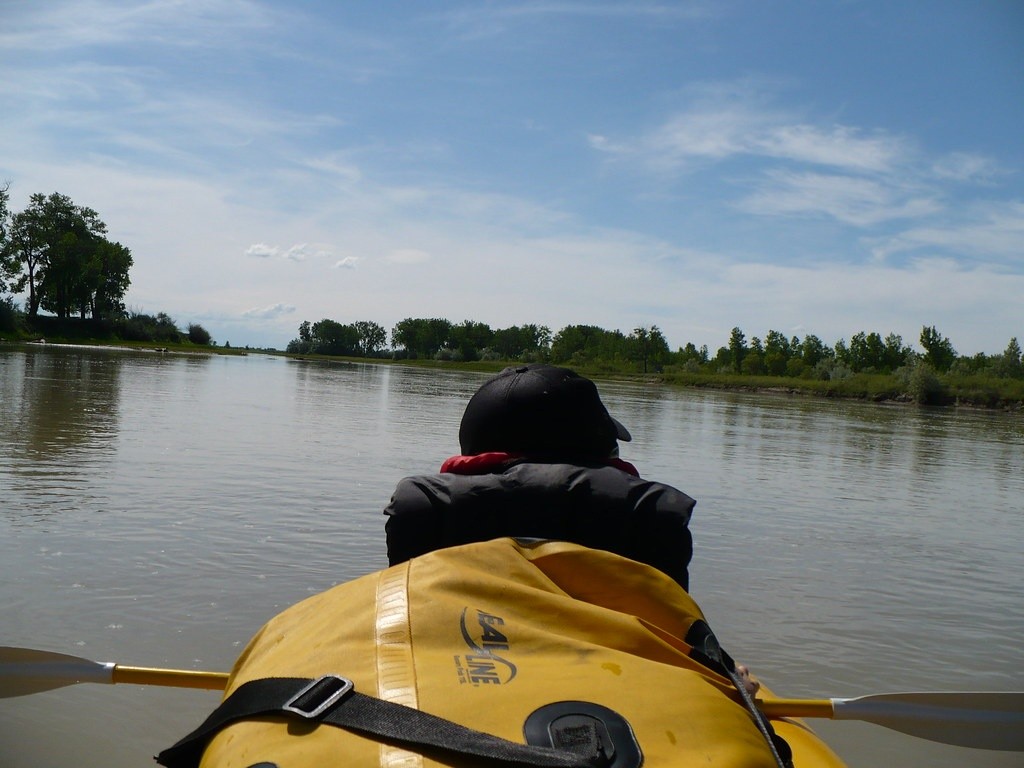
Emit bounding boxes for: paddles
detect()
[0,644,1024,756]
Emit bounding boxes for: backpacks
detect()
[152,532,785,768]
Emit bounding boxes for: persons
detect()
[387,365,696,595]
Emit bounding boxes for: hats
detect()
[458,365,632,467]
